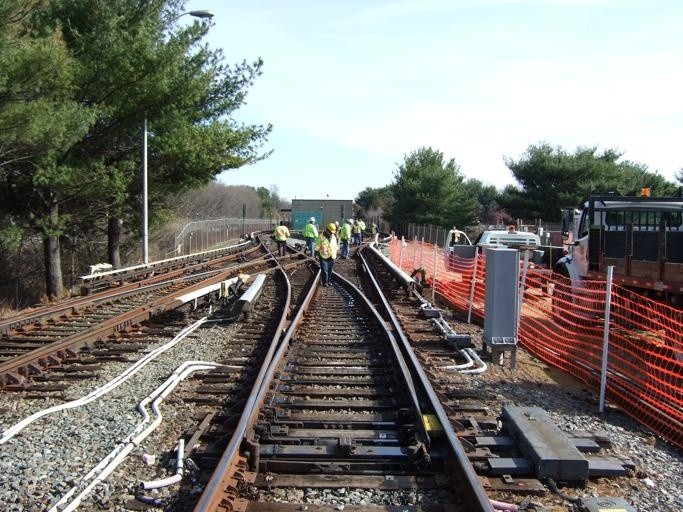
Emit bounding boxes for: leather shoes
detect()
[323,283,328,287]
[328,282,332,286]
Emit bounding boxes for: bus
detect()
[550,191,682,325]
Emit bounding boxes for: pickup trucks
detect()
[442,224,566,299]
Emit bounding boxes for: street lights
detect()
[139,9,214,265]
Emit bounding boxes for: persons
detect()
[450,225,461,255]
[271,216,382,287]
[507,225,519,248]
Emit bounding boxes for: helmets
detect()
[346,218,353,224]
[309,216,315,222]
[326,223,336,234]
